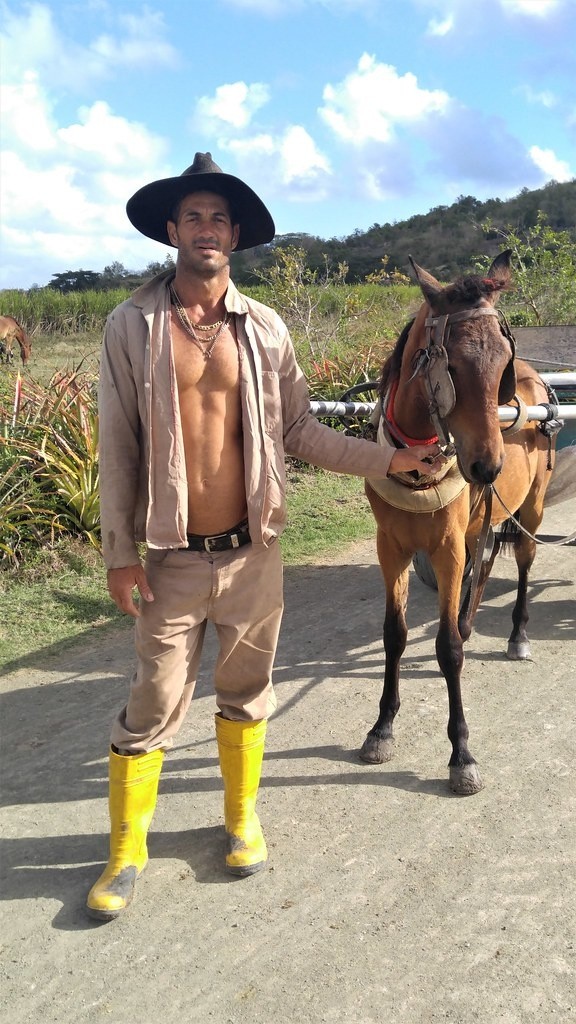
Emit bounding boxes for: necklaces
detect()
[168,282,234,360]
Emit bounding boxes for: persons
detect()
[83,149,448,926]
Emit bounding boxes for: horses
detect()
[357,248,556,797]
[0,315,32,366]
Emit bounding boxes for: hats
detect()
[126,152,276,249]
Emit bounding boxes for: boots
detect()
[85,743,165,920]
[212,712,269,876]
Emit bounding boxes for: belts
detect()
[174,519,251,553]
